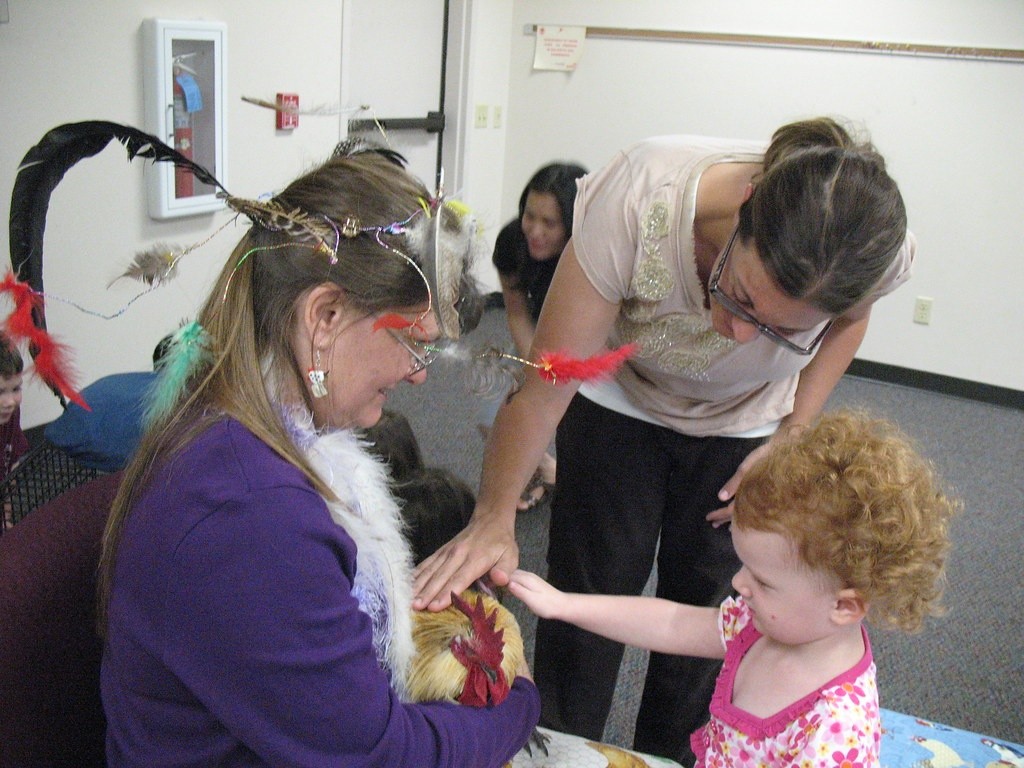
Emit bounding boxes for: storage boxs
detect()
[142,16,230,223]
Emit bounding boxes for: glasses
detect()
[365,306,439,378]
[708,223,836,353]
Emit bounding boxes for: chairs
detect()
[0,470,130,768]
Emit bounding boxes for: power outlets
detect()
[914,296,933,326]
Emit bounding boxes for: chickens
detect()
[405,582,551,759]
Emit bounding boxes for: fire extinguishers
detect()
[173,52,203,199]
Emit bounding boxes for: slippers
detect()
[514,478,559,515]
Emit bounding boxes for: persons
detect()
[0,331,477,768]
[483,407,952,768]
[0,330,30,484]
[91,150,683,768]
[407,117,917,768]
[493,163,589,512]
